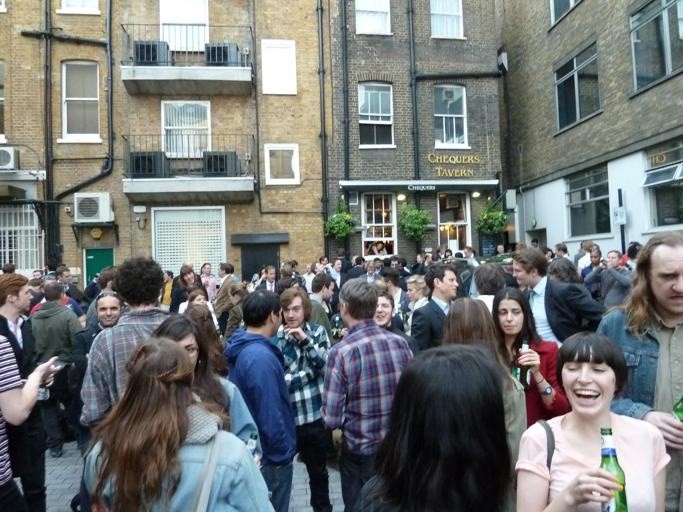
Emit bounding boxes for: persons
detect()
[0,234,683,512]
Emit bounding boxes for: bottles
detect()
[672,396,683,422]
[517,339,532,391]
[37,381,50,401]
[600,256,605,263]
[333,315,341,339]
[247,433,258,456]
[597,427,628,511]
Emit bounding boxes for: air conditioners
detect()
[203,152,241,176]
[74,192,115,222]
[133,40,171,66]
[128,151,169,178]
[205,44,242,66]
[0,147,18,170]
